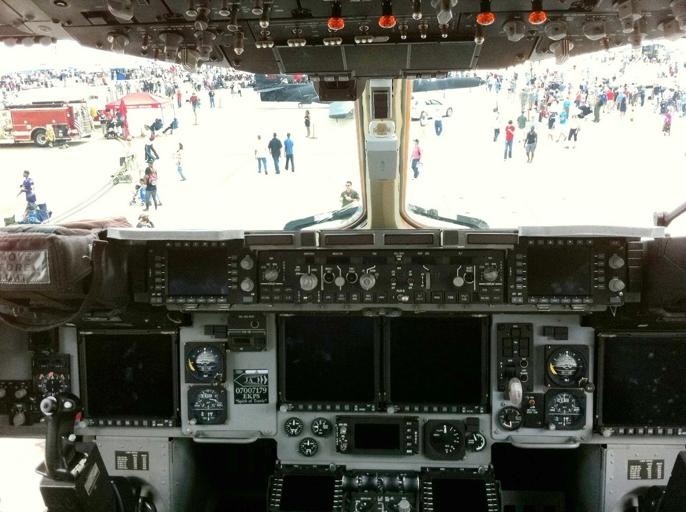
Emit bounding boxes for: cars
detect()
[409,93,454,127]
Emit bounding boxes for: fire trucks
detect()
[0,94,92,148]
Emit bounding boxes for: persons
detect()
[284,133,295,172]
[123,125,161,210]
[448,53,686,163]
[339,180,358,209]
[304,110,312,138]
[411,138,423,179]
[17,171,36,205]
[268,133,281,174]
[253,135,268,175]
[434,109,443,136]
[136,214,154,228]
[0,63,257,141]
[177,142,187,181]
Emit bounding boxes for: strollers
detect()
[111,153,137,184]
